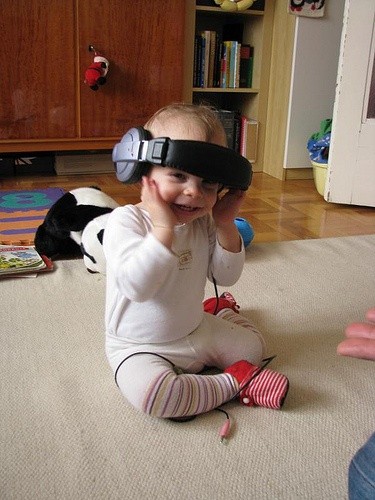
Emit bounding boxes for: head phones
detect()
[112,127,252,191]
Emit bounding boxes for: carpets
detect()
[1,235,375,500]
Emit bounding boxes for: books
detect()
[214,107,259,163]
[0,244,53,279]
[194,29,254,88]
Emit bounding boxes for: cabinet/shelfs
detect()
[0,0,275,172]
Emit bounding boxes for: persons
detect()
[103,103,291,419]
[337,310,375,500]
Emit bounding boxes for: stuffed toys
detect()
[84,53,110,92]
[35,185,122,275]
